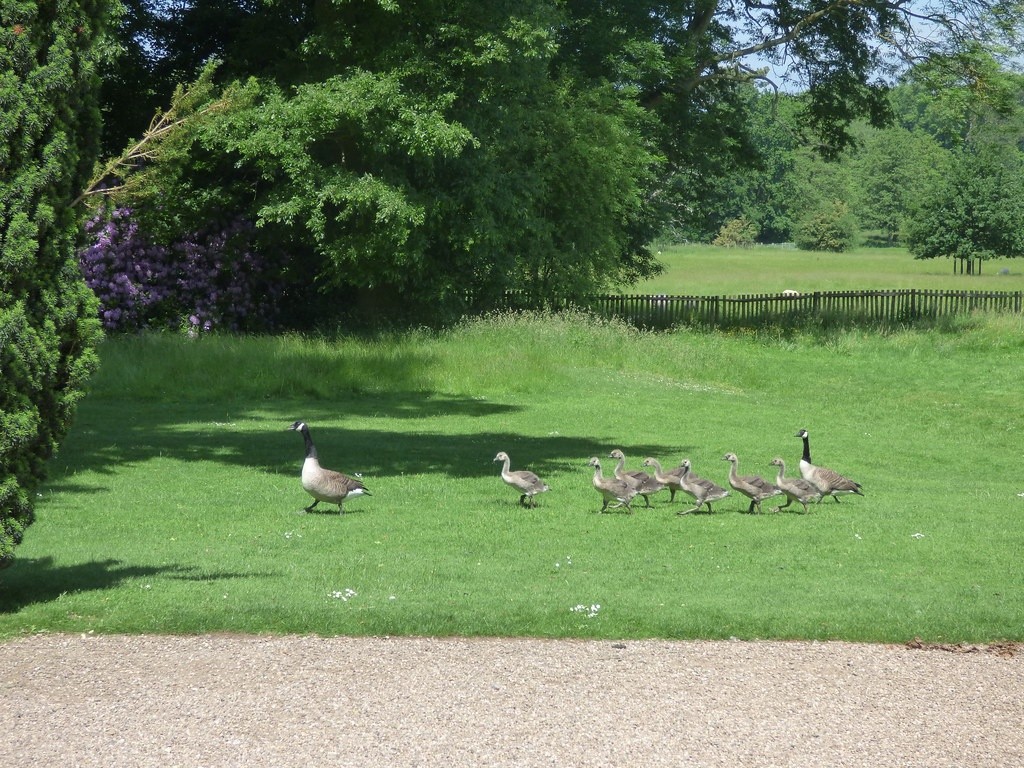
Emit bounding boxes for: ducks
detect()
[587,457,638,516]
[492,451,549,506]
[607,449,823,516]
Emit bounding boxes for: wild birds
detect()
[793,429,864,505]
[286,420,372,515]
